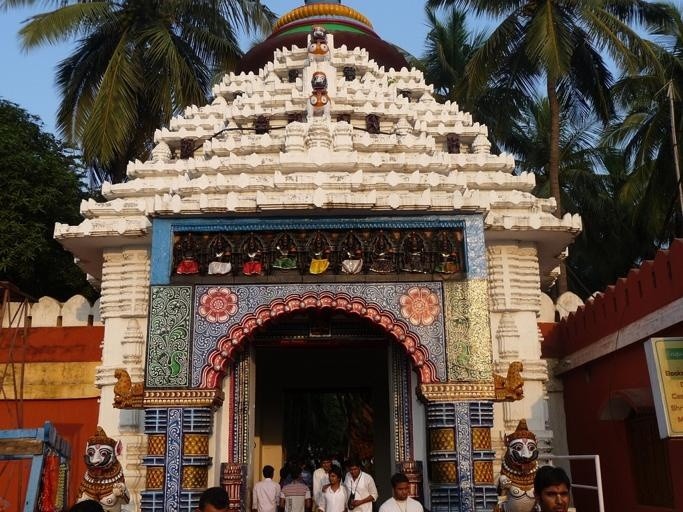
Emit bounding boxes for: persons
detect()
[533,465,571,511]
[197,451,424,512]
[69,498,104,511]
